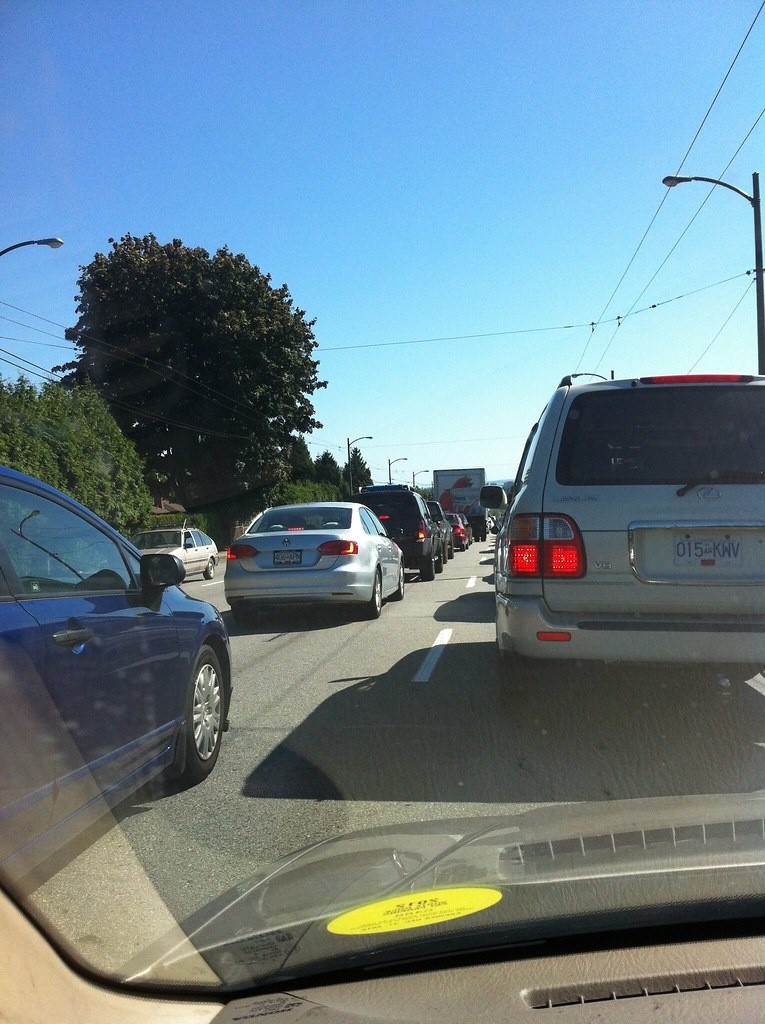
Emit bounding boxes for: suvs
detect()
[479,373,765,703]
[347,484,444,582]
[426,500,455,564]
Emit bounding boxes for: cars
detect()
[445,512,472,551]
[130,518,219,579]
[0,465,235,891]
[223,502,406,629]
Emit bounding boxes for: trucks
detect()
[432,468,486,542]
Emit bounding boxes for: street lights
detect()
[388,457,408,485]
[413,470,430,487]
[347,436,373,499]
[663,172,765,377]
[0,237,64,257]
